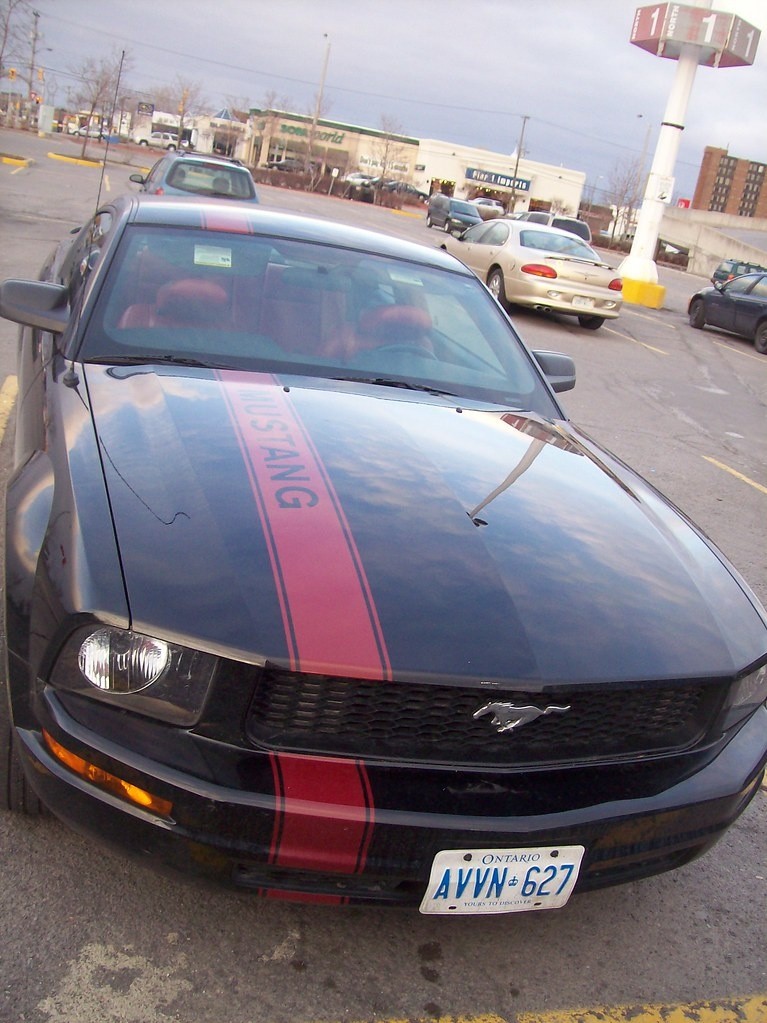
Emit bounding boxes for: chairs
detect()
[173,168,192,188]
[213,177,230,193]
[316,305,433,360]
[117,278,249,332]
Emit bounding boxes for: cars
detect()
[72,125,112,141]
[686,269,767,355]
[129,150,259,206]
[468,198,637,250]
[337,170,429,204]
[440,219,625,330]
[1,190,767,917]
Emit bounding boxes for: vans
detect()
[710,259,766,285]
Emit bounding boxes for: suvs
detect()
[426,193,484,235]
[134,131,194,153]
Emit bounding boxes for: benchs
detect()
[123,249,347,352]
